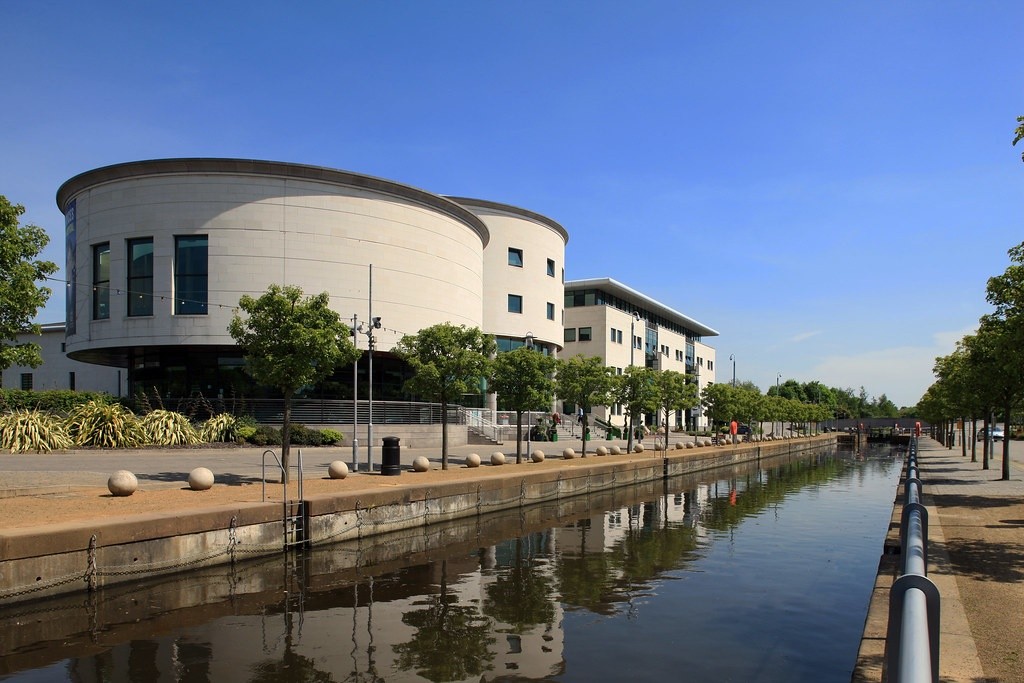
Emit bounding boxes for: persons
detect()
[576,405,583,425]
[662,418,666,427]
[553,413,562,425]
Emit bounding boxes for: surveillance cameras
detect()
[372,317,381,322]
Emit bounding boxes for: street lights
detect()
[777,372,782,396]
[631,311,640,366]
[730,354,735,386]
[522,331,538,460]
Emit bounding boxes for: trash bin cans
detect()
[380,435,403,478]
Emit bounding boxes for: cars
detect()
[977,426,1004,442]
